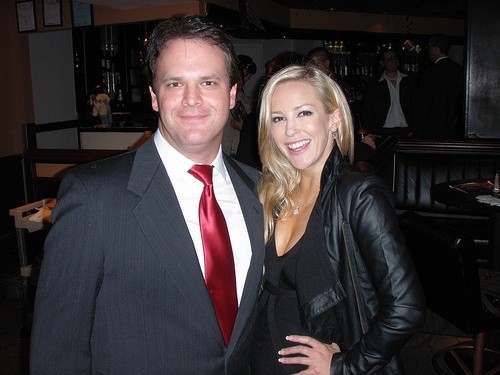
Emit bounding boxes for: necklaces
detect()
[293,184,320,215]
[387,74,398,80]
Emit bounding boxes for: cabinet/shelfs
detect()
[324,38,432,107]
[75,19,158,118]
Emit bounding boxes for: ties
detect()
[188,164,238,349]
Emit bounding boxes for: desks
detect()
[9,196,56,292]
[431,177,496,210]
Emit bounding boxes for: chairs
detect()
[397,211,500,375]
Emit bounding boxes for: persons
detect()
[29,16,426,375]
[414,32,466,140]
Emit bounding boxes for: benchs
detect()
[375,136,500,269]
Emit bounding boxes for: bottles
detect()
[354,127,375,174]
[101,34,149,112]
[325,42,423,113]
